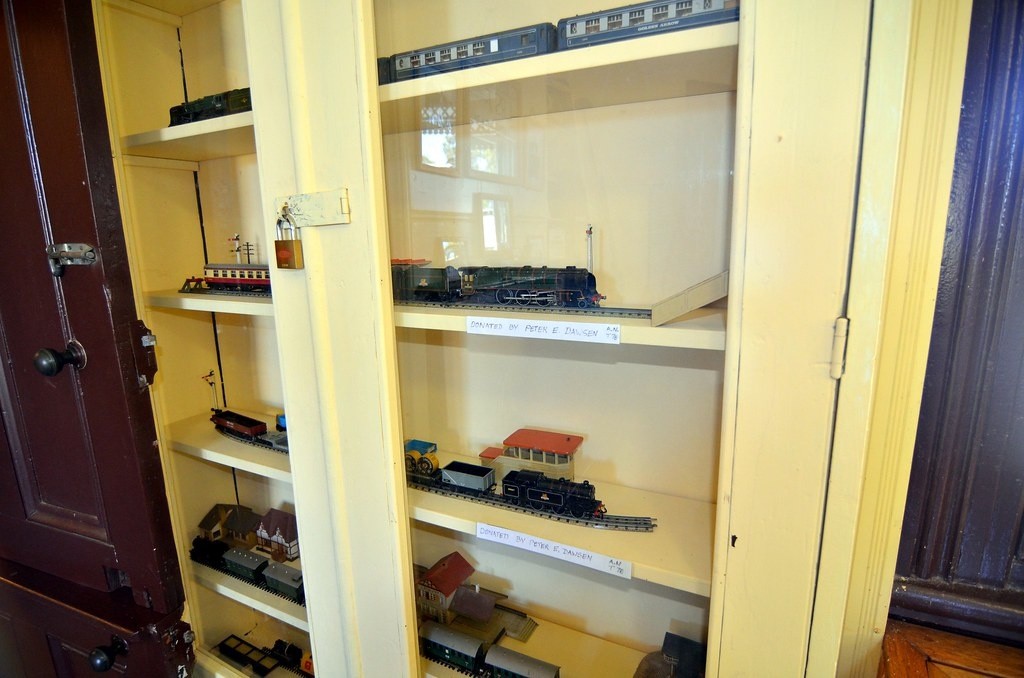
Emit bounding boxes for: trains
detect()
[167,0,740,132]
[202,368,607,521]
[203,225,608,310]
[190,533,562,678]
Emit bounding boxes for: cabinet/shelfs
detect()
[88,0,919,678]
